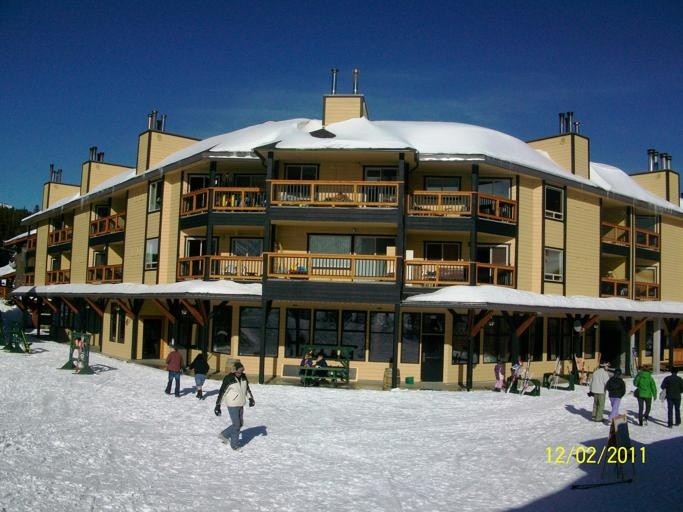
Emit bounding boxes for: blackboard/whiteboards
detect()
[612,414,635,462]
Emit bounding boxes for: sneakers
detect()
[236,447,243,452]
[592,416,604,422]
[218,433,230,446]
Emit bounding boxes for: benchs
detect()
[298,365,345,388]
[505,369,593,397]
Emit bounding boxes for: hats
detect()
[231,362,245,373]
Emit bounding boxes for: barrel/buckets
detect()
[276,191,287,206]
[405,376,414,384]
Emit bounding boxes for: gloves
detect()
[214,405,221,416]
[249,398,255,407]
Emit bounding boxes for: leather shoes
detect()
[639,413,648,426]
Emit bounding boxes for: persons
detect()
[631,364,659,427]
[310,353,328,388]
[511,354,524,379]
[163,346,185,398]
[659,365,682,429]
[297,353,314,386]
[604,368,626,426]
[184,352,209,400]
[210,362,256,454]
[587,361,610,422]
[493,360,506,392]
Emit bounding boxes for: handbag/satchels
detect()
[659,389,667,401]
[634,389,639,397]
